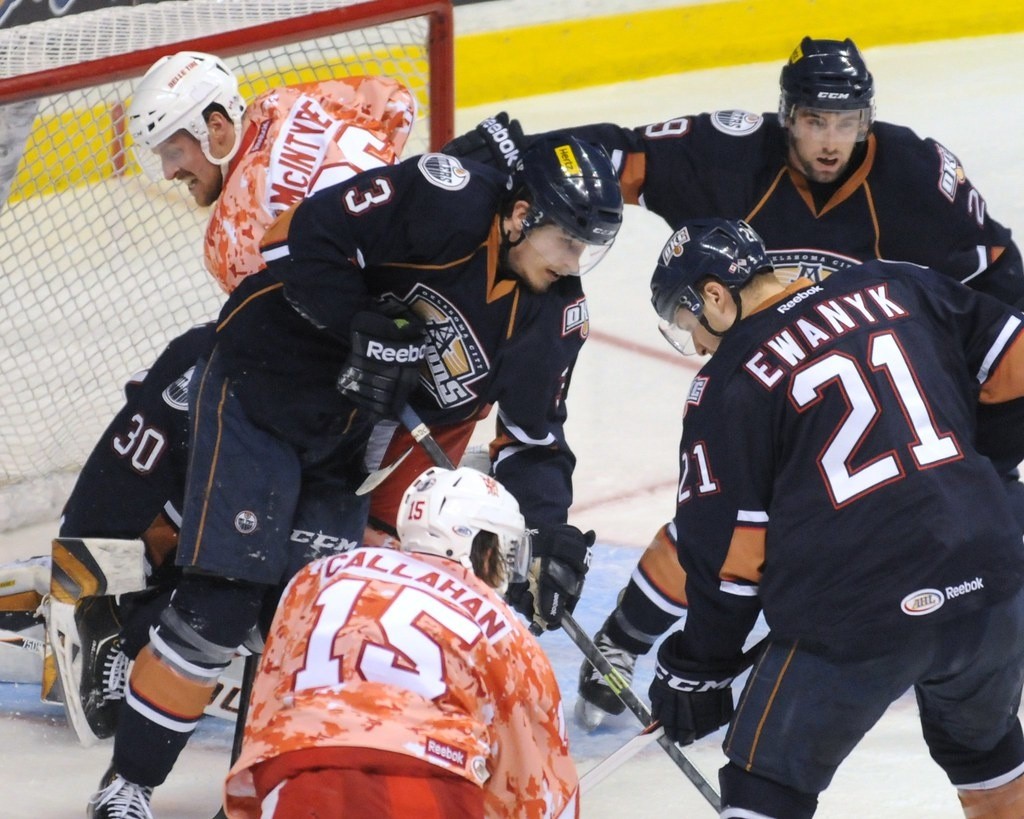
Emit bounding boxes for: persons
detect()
[127,52,493,550]
[30,108,626,819]
[212,467,581,819]
[642,210,1024,817]
[576,30,1024,727]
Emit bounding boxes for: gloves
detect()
[337,298,432,442]
[644,632,743,749]
[507,512,593,632]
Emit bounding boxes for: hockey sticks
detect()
[396,396,728,819]
[577,628,778,792]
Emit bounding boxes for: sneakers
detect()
[575,612,636,731]
[87,766,154,818]
[75,588,133,738]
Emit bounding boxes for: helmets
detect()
[394,460,527,600]
[648,222,773,353]
[504,134,625,283]
[114,55,255,185]
[778,33,877,149]
